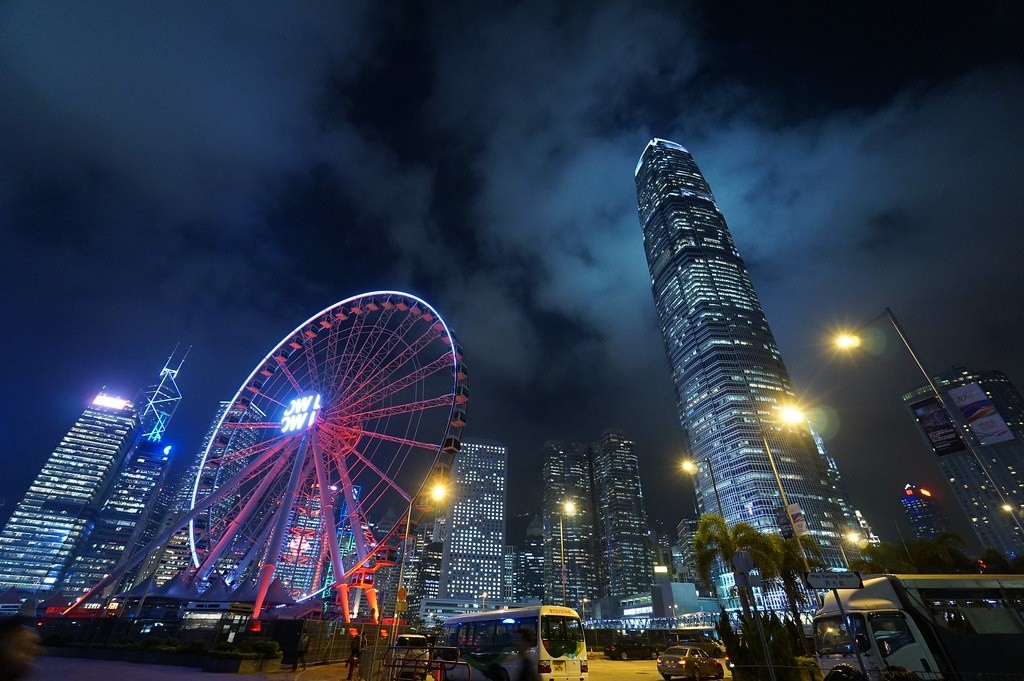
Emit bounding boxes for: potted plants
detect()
[203,640,283,673]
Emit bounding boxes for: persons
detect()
[345,630,368,668]
[882,618,913,654]
[0,615,41,681]
[515,628,543,681]
[288,629,309,672]
[833,630,851,653]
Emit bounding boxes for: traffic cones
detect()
[589,647,595,657]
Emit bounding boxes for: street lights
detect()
[680,457,751,625]
[834,306,1024,533]
[761,405,821,607]
[391,485,446,645]
[560,503,575,640]
[482,592,487,613]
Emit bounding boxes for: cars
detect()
[657,646,724,681]
[604,640,659,661]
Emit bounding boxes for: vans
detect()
[385,635,431,679]
[665,632,723,658]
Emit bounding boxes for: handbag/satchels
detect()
[306,644,312,653]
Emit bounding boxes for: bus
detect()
[431,605,589,681]
[813,573,1024,681]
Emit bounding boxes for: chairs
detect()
[477,633,582,647]
[449,633,460,647]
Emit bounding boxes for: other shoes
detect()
[345,664,347,667]
[292,670,295,672]
[303,668,306,671]
[354,664,358,668]
